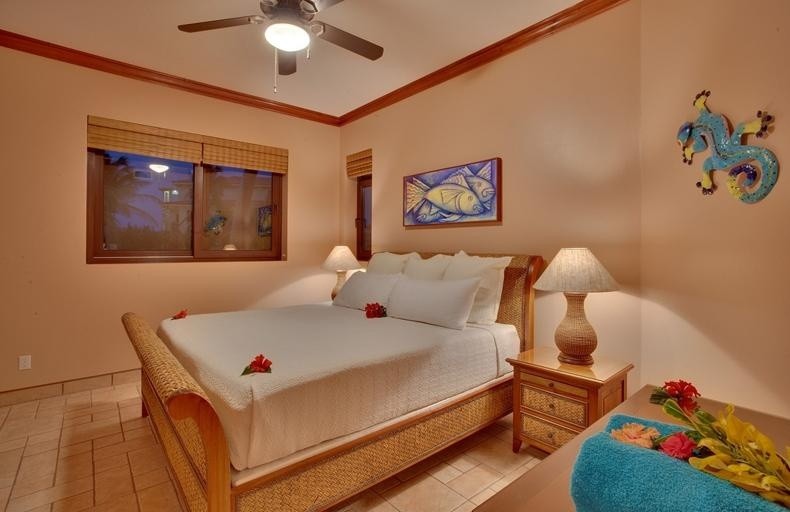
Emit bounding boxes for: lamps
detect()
[532,247,620,366]
[263,13,310,60]
[322,246,361,301]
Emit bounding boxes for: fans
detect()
[161,1,384,96]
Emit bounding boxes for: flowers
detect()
[171,307,188,320]
[365,302,386,318]
[240,354,272,376]
[613,379,790,510]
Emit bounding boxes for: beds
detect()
[120,253,538,510]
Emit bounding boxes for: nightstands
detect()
[504,343,634,454]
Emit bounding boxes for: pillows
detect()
[330,250,514,330]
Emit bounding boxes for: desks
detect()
[469,384,790,511]
